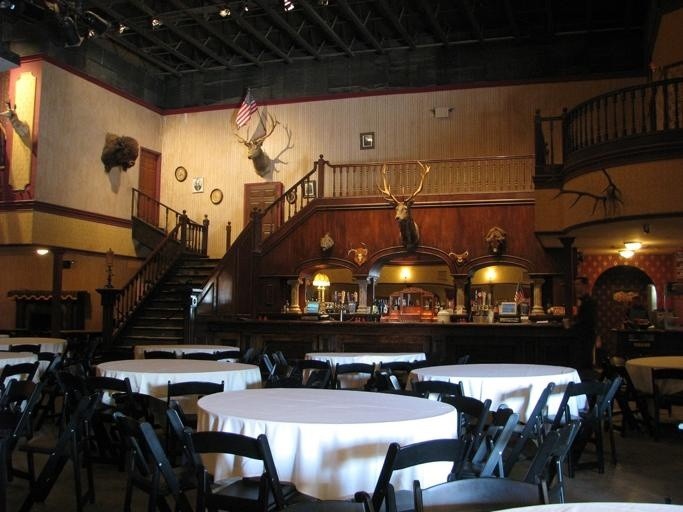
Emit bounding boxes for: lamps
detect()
[313,269,329,301]
[618,247,633,259]
[622,241,641,252]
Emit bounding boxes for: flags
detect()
[234,87,258,128]
[512,280,525,303]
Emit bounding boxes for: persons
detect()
[562,277,603,371]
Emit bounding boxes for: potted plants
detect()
[99,133,138,189]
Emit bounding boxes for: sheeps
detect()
[346,247,369,267]
[447,250,470,268]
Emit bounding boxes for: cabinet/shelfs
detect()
[387,285,438,320]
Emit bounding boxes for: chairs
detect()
[0,345,623,512]
[593,329,683,433]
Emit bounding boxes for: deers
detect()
[376,159,432,248]
[0,100,29,138]
[233,105,282,174]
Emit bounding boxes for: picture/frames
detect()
[302,179,315,199]
[173,165,187,182]
[358,130,374,151]
[210,189,224,205]
[285,192,296,204]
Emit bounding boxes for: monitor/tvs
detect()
[500,301,517,316]
[307,302,320,312]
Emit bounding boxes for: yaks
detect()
[101,133,138,171]
